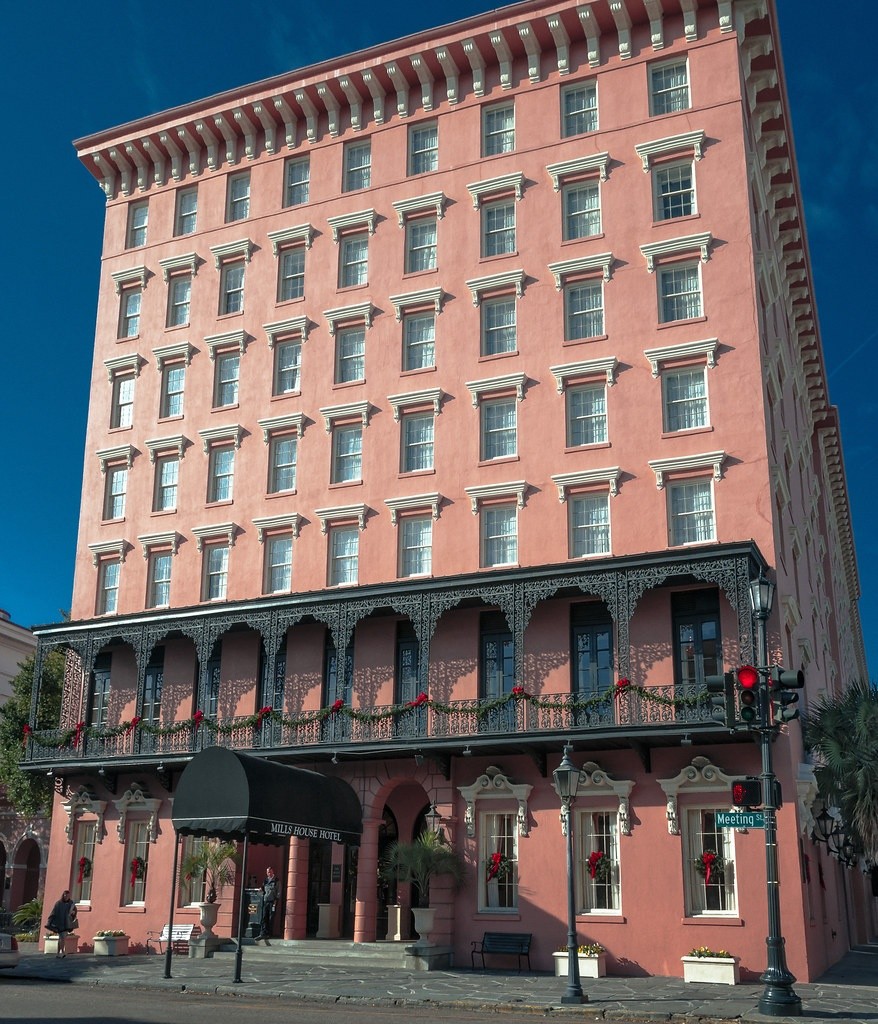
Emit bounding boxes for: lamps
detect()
[811,801,859,870]
[157,761,164,772]
[564,740,574,751]
[681,732,692,749]
[463,745,472,758]
[99,767,105,776]
[47,768,53,777]
[425,800,444,845]
[332,752,337,764]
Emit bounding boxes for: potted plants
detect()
[178,839,241,939]
[382,828,472,947]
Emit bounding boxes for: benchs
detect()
[471,931,532,974]
[147,924,195,956]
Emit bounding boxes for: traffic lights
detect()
[735,665,758,723]
[771,666,805,725]
[705,673,735,728]
[730,779,762,807]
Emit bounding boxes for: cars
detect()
[0,932,19,970]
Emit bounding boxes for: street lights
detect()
[551,747,589,1005]
[748,566,803,1017]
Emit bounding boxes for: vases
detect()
[552,952,607,978]
[681,956,741,985]
[44,935,80,954]
[92,936,131,955]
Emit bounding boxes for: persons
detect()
[255,866,280,940]
[44,891,79,959]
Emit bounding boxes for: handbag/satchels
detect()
[72,919,78,928]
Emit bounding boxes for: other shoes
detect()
[56,955,62,958]
[255,934,265,940]
[62,954,66,958]
[264,934,270,939]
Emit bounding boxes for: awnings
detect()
[171,746,363,847]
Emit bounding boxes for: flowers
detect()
[96,930,126,937]
[46,931,74,939]
[689,946,730,958]
[558,942,606,957]
